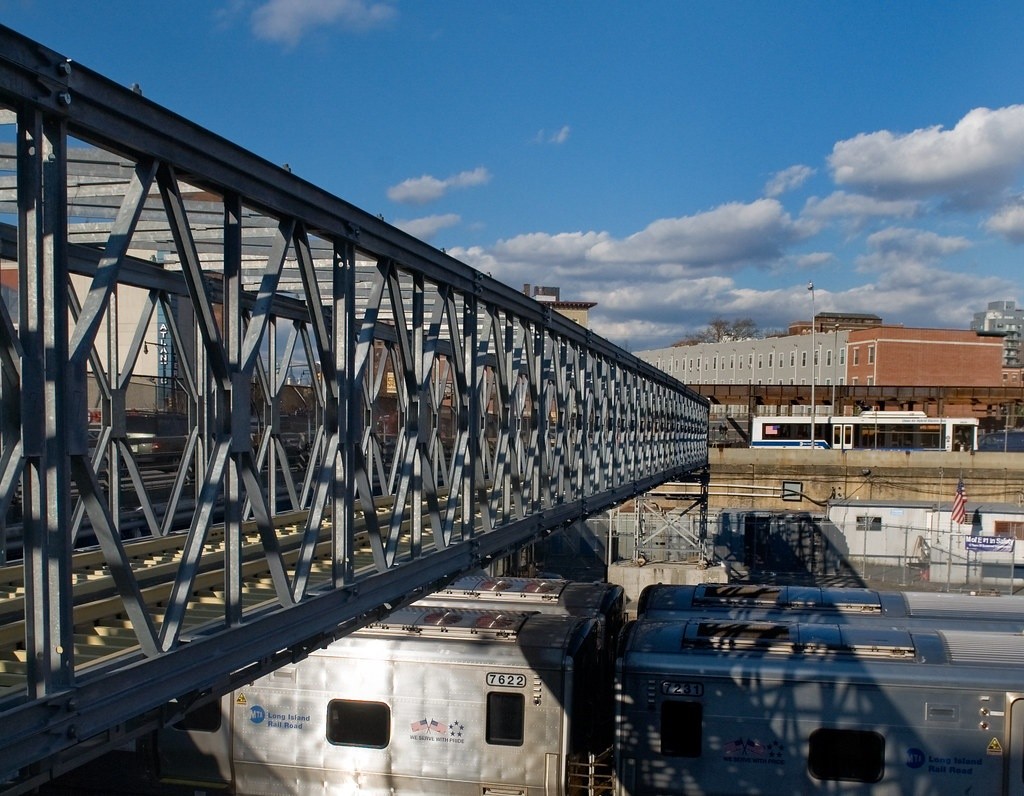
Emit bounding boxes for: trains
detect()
[51,566,1023,796]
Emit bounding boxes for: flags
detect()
[951,477,968,524]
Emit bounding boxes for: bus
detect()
[749,409,980,454]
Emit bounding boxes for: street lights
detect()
[807,280,816,450]
